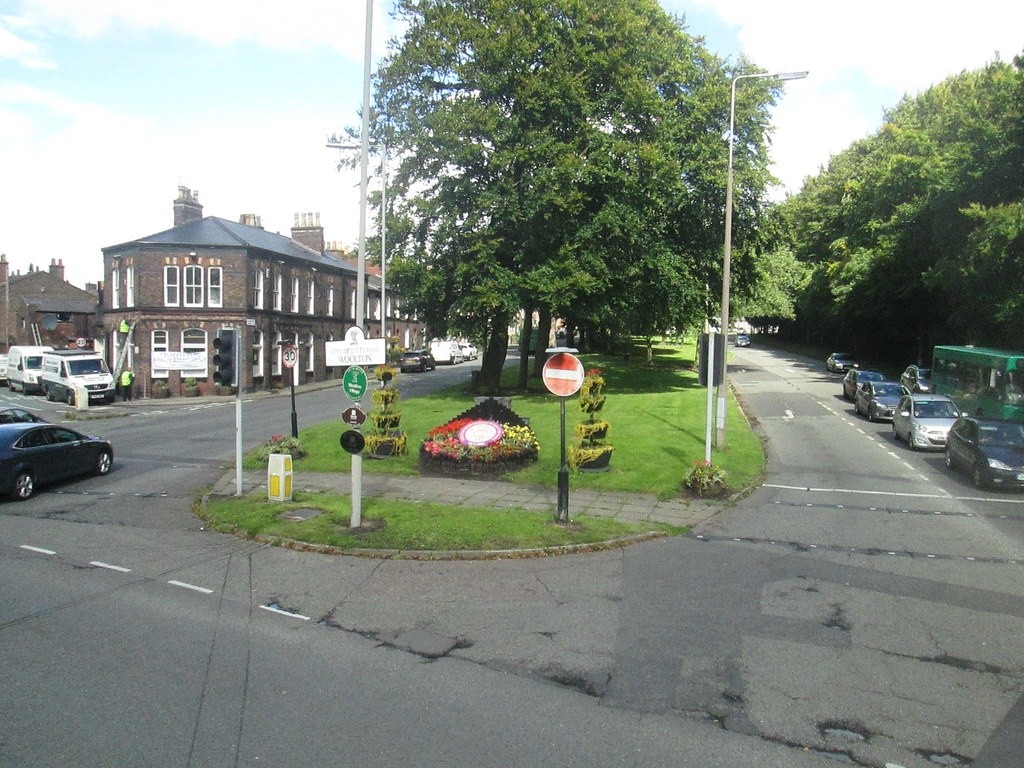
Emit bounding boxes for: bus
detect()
[932,345,1024,425]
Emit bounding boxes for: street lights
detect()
[917,269,933,366]
[714,72,809,451]
[326,143,385,411]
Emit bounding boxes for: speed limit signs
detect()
[281,345,298,368]
[76,338,87,348]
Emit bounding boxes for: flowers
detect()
[682,458,728,498]
[213,382,236,392]
[580,369,604,404]
[258,432,307,463]
[365,427,408,457]
[153,379,170,389]
[374,364,398,381]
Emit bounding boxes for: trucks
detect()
[519,325,539,354]
[41,350,117,406]
[6,345,54,395]
[430,340,464,364]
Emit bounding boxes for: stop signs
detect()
[542,353,585,397]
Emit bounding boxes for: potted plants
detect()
[369,385,402,429]
[567,396,615,475]
[183,378,199,397]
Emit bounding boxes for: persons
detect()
[119,367,134,401]
[119,314,133,351]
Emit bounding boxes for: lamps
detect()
[190,252,197,260]
[113,255,123,260]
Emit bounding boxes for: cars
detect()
[826,352,861,373]
[944,417,1024,491]
[735,336,751,347]
[842,369,887,402]
[893,394,968,450]
[853,381,910,421]
[0,407,113,501]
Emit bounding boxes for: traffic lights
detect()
[213,327,239,387]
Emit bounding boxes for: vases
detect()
[218,388,232,396]
[695,481,723,496]
[376,372,392,381]
[588,381,598,393]
[291,448,303,459]
[370,442,400,456]
[152,388,169,398]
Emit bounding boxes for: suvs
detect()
[400,348,435,373]
[458,342,478,361]
[900,365,932,394]
